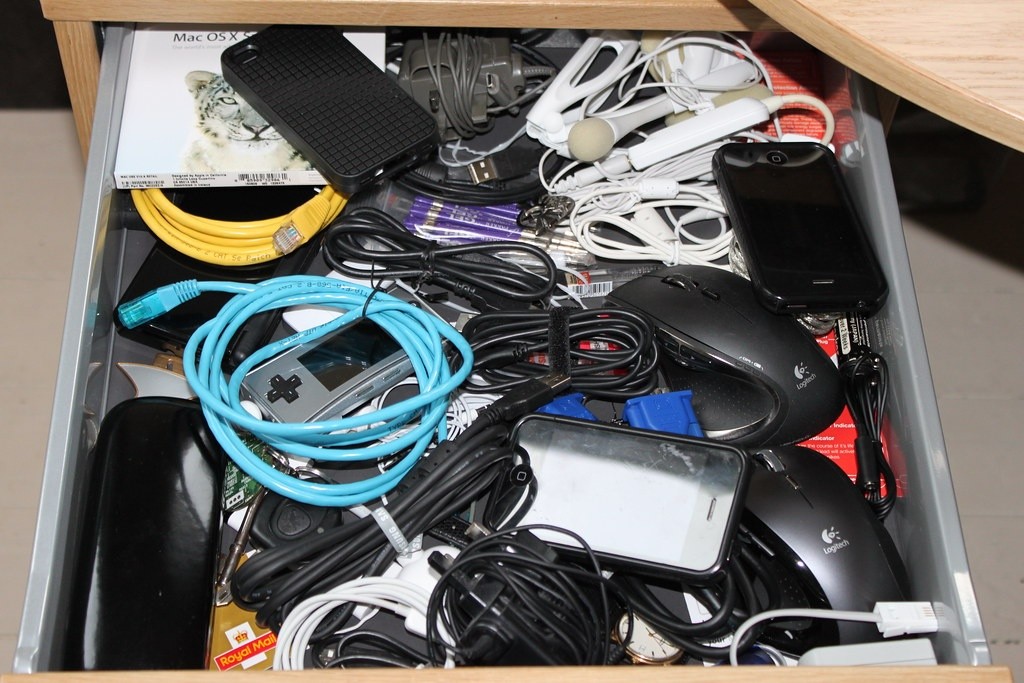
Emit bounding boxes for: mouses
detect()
[734,444,912,659]
[599,264,846,451]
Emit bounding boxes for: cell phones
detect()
[483,412,755,588]
[711,140,888,317]
[112,186,329,374]
[220,25,440,192]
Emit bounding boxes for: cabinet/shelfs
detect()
[0,1,1023,682]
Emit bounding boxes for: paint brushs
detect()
[405,197,595,266]
